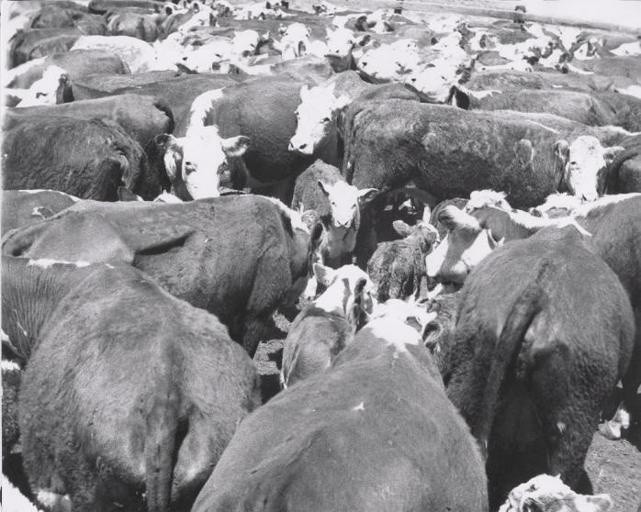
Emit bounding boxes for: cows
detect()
[0,0,641,511]
